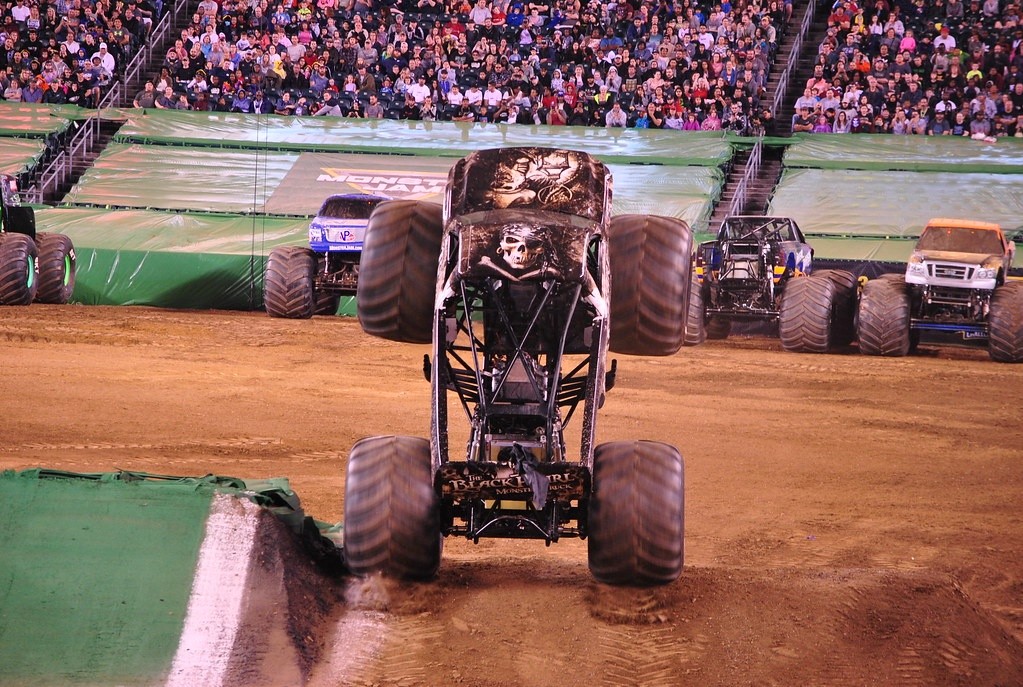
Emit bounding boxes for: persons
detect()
[133,0,801,137]
[0,0,169,109]
[791,0,1023,140]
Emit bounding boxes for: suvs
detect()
[850,219,1023,362]
[0,173,76,305]
[344,142,691,586]
[683,215,854,355]
[264,190,390,319]
